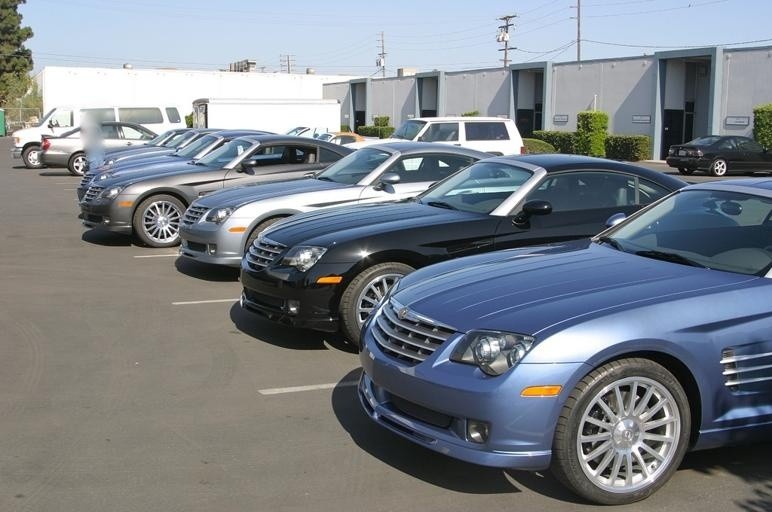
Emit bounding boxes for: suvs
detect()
[339,116,525,160]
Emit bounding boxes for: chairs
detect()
[420,156,440,171]
[279,146,315,163]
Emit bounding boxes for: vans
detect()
[10,101,190,170]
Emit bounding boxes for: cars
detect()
[176,142,499,271]
[239,153,689,352]
[37,122,162,177]
[74,126,365,249]
[355,177,772,504]
[667,134,771,178]
